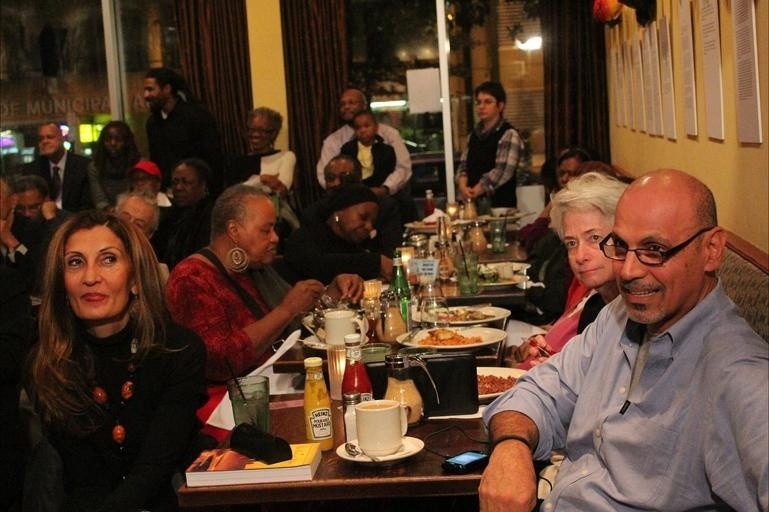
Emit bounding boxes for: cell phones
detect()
[442,451,488,473]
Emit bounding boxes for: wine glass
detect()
[421,280,441,298]
[419,296,450,354]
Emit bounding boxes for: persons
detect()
[504,144,634,375]
[454,78,522,210]
[476,167,769,511]
[1,68,412,381]
[18,208,216,511]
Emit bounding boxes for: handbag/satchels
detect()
[367,354,479,419]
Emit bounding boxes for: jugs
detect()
[375,291,407,344]
[464,222,487,252]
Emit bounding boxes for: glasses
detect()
[599,226,712,267]
[471,97,500,104]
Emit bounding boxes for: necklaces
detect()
[71,321,146,485]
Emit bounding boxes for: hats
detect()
[124,161,162,177]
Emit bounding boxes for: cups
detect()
[488,218,507,253]
[227,375,271,436]
[457,253,478,294]
[323,311,367,346]
[355,399,405,456]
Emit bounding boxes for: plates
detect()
[397,325,506,350]
[488,262,531,272]
[303,332,369,348]
[479,273,530,286]
[475,365,529,399]
[336,437,424,462]
[479,207,531,220]
[411,305,511,324]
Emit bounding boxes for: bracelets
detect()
[488,433,533,456]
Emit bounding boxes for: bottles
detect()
[340,333,373,450]
[302,357,336,453]
[391,248,411,325]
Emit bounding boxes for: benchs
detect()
[592,158,768,350]
